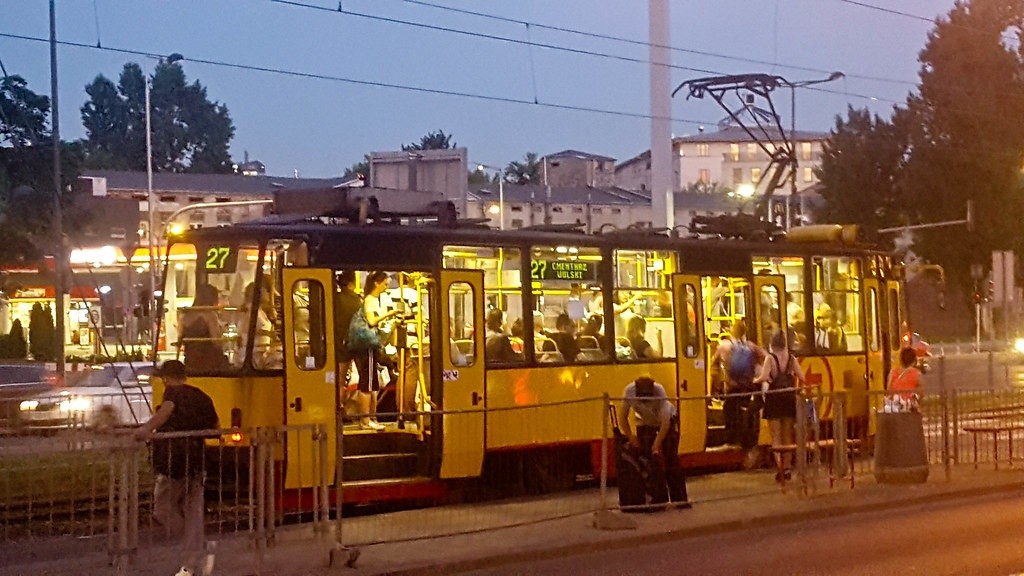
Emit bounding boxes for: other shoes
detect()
[723,442,741,451]
[359,419,386,431]
[775,468,792,483]
[174,566,193,576]
[649,497,668,505]
[201,540,217,576]
[676,499,694,512]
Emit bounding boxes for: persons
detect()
[888,347,921,400]
[627,317,661,359]
[133,360,221,576]
[701,276,729,340]
[422,318,465,393]
[178,283,228,370]
[543,314,586,362]
[713,320,768,471]
[575,314,610,353]
[234,283,280,367]
[568,290,641,337]
[619,376,687,503]
[486,307,515,361]
[533,309,547,351]
[753,330,806,480]
[761,290,847,351]
[334,270,404,429]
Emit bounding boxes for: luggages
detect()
[608,403,667,513]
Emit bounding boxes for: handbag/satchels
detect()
[346,296,382,353]
[376,362,391,387]
[344,359,360,391]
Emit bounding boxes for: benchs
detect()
[961,405,1024,472]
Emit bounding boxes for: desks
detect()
[768,437,859,497]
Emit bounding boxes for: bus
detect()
[148,70,915,525]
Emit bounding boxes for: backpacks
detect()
[721,336,756,382]
[766,352,797,408]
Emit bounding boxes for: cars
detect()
[0,359,161,435]
[908,330,936,374]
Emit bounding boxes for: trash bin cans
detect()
[873,411,929,483]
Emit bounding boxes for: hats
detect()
[534,310,544,326]
[152,360,187,378]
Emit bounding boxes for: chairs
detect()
[458,336,640,359]
